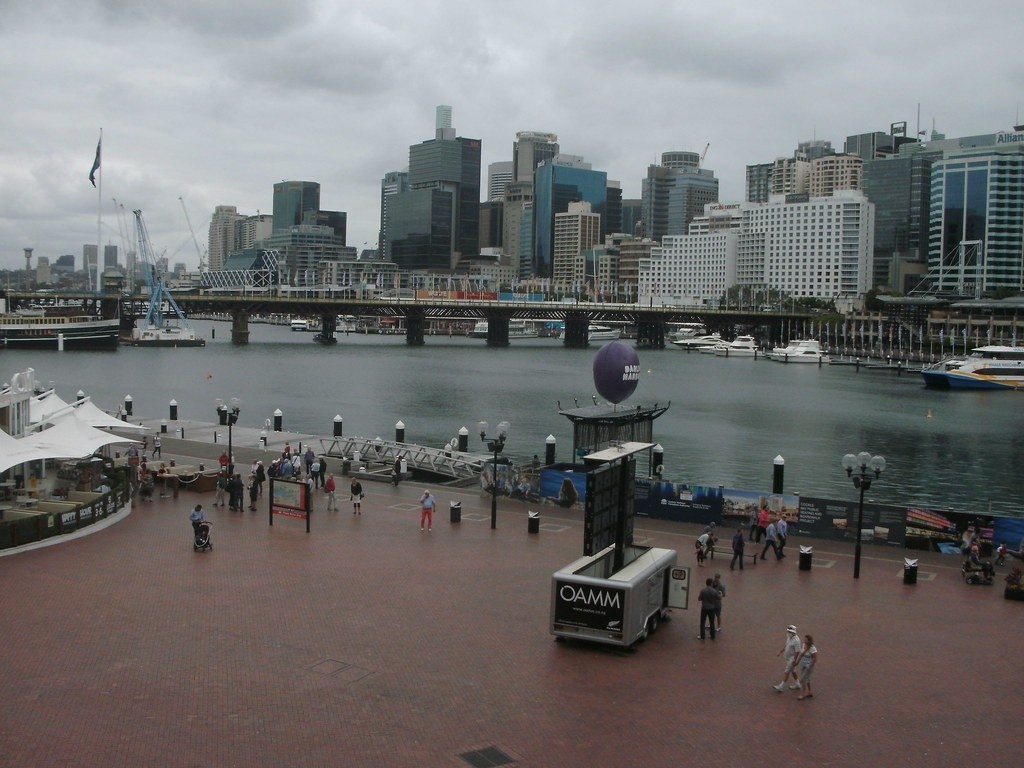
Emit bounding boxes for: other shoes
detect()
[698,561,705,567]
[152,454,154,458]
[353,511,356,514]
[776,556,783,560]
[421,528,424,530]
[755,542,760,544]
[797,696,806,700]
[328,508,332,511]
[334,508,339,511]
[781,554,786,557]
[789,684,800,689]
[320,487,324,489]
[805,694,813,697]
[773,686,784,692]
[749,538,753,541]
[212,501,258,513]
[428,528,432,531]
[760,556,767,560]
[358,511,361,515]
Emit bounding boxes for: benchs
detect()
[710,547,757,564]
[2,490,105,522]
[112,457,223,493]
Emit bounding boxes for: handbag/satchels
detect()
[324,484,330,493]
[260,474,265,481]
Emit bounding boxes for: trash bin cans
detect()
[528,510,540,533]
[450,500,462,522]
[799,544,813,570]
[903,557,919,584]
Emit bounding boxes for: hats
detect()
[786,625,796,634]
[257,461,262,464]
[768,517,779,522]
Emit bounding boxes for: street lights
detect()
[842,451,886,578]
[477,421,511,530]
[216,398,240,475]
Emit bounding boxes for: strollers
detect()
[193,521,214,552]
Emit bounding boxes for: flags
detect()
[89,139,101,187]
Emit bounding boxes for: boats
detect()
[558,323,621,340]
[672,328,765,357]
[766,340,831,363]
[468,319,539,339]
[921,345,1024,391]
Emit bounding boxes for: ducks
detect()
[927,409,931,419]
[648,368,651,372]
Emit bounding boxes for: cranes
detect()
[112,197,204,331]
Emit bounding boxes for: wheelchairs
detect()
[965,560,992,585]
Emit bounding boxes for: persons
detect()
[350,477,362,514]
[77,431,167,503]
[760,514,787,560]
[213,449,266,512]
[189,505,207,542]
[695,522,717,566]
[962,528,982,559]
[698,573,726,639]
[730,528,743,569]
[115,401,123,418]
[749,505,770,541]
[482,472,529,499]
[995,543,1007,566]
[325,474,338,511]
[420,490,435,531]
[968,545,992,581]
[532,455,540,468]
[391,456,404,486]
[267,441,326,512]
[774,624,817,699]
